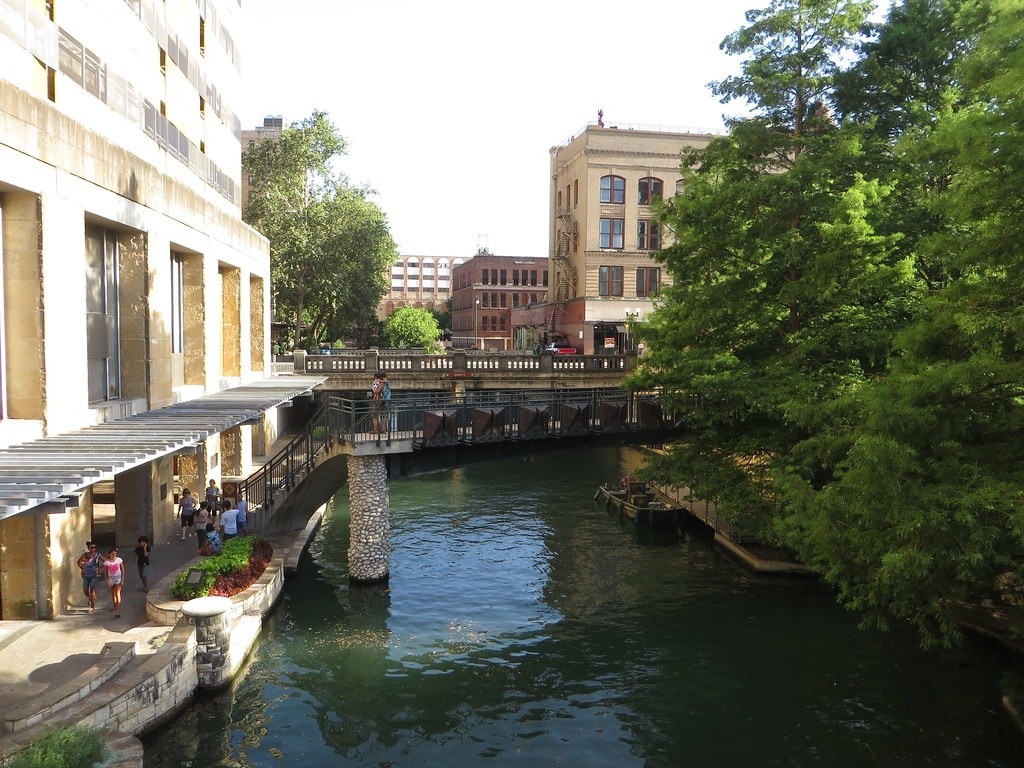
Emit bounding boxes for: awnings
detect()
[271,322,306,328]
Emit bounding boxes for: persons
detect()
[177,488,198,540]
[371,373,387,400]
[273,341,287,354]
[205,479,221,527]
[132,536,151,593]
[198,523,221,555]
[77,541,106,613]
[195,501,208,552]
[369,372,391,434]
[219,500,239,541]
[103,548,124,616]
[81,554,101,577]
[237,493,248,536]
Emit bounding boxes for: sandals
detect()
[369,429,378,434]
[380,430,386,434]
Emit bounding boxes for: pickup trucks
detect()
[547,341,577,360]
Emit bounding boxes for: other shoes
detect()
[88,598,91,606]
[188,533,192,538]
[112,606,118,610]
[179,536,185,541]
[87,608,95,613]
[115,611,120,617]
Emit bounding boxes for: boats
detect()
[599,478,685,531]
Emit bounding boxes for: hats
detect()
[206,523,215,532]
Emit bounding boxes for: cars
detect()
[318,345,330,354]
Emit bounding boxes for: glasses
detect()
[184,491,191,495]
[90,546,96,549]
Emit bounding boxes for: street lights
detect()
[474,297,480,349]
